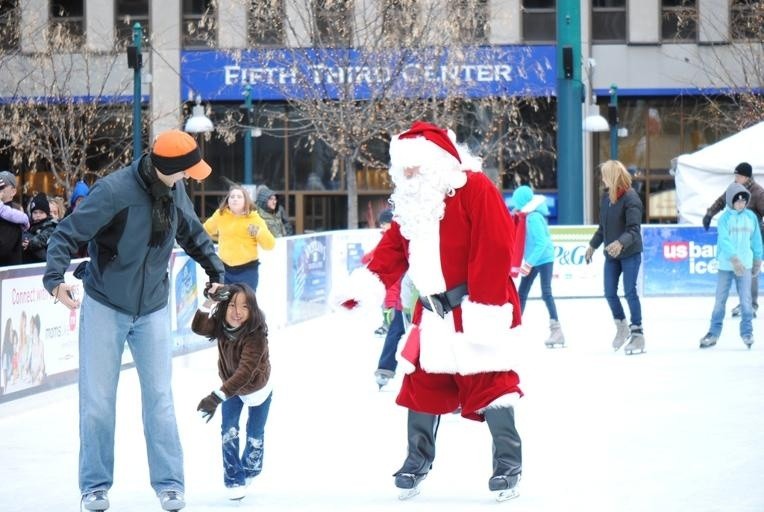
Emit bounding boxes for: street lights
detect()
[185,94,215,224]
[583,95,611,225]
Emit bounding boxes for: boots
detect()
[623,325,645,349]
[546,322,564,345]
[613,319,629,349]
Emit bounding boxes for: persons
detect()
[512,185,564,344]
[699,182,763,345]
[191,282,272,501]
[373,208,406,390]
[585,160,645,350]
[702,163,764,316]
[327,121,525,490]
[202,175,275,293]
[42,129,231,511]
[0,170,89,267]
[255,183,293,238]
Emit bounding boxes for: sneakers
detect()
[752,308,756,317]
[376,373,390,386]
[732,304,741,314]
[375,328,388,335]
[83,492,109,511]
[159,490,185,511]
[227,487,247,500]
[700,332,717,346]
[490,472,521,490]
[744,333,754,345]
[396,473,427,489]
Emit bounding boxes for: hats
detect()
[378,209,394,223]
[733,192,748,205]
[389,122,466,190]
[0,172,16,188]
[512,186,533,210]
[30,191,50,217]
[150,128,212,180]
[734,162,752,177]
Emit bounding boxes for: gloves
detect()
[197,389,228,423]
[703,214,711,230]
[752,260,761,276]
[204,283,232,301]
[519,263,533,277]
[607,240,624,258]
[586,245,596,263]
[729,256,745,276]
